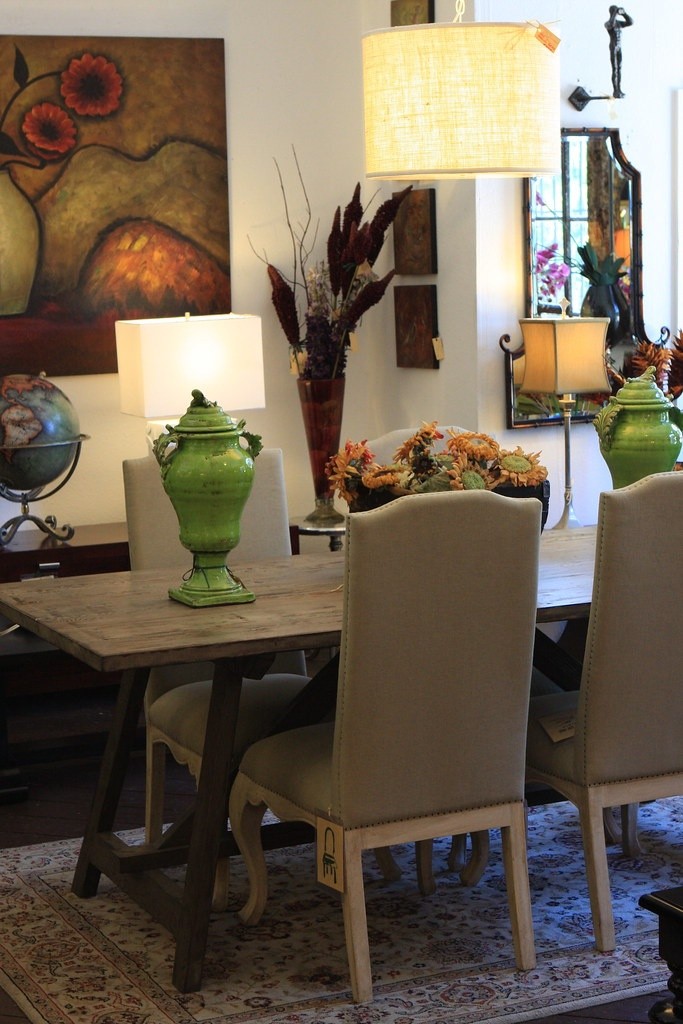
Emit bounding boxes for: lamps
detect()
[517,318,613,530]
[362,0,563,182]
[114,313,265,450]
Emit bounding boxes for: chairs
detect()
[454,471,683,956]
[228,491,543,1000]
[122,448,318,908]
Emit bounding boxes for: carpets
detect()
[0,795,683,1024]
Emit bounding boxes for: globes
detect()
[0,370,92,548]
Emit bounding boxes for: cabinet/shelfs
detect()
[0,521,169,803]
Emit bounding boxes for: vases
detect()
[581,285,628,350]
[297,374,346,521]
[347,481,550,535]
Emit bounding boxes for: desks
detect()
[0,526,597,991]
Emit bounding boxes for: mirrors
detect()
[499,126,677,429]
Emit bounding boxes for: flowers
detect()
[248,151,416,376]
[325,421,549,505]
[533,191,631,296]
